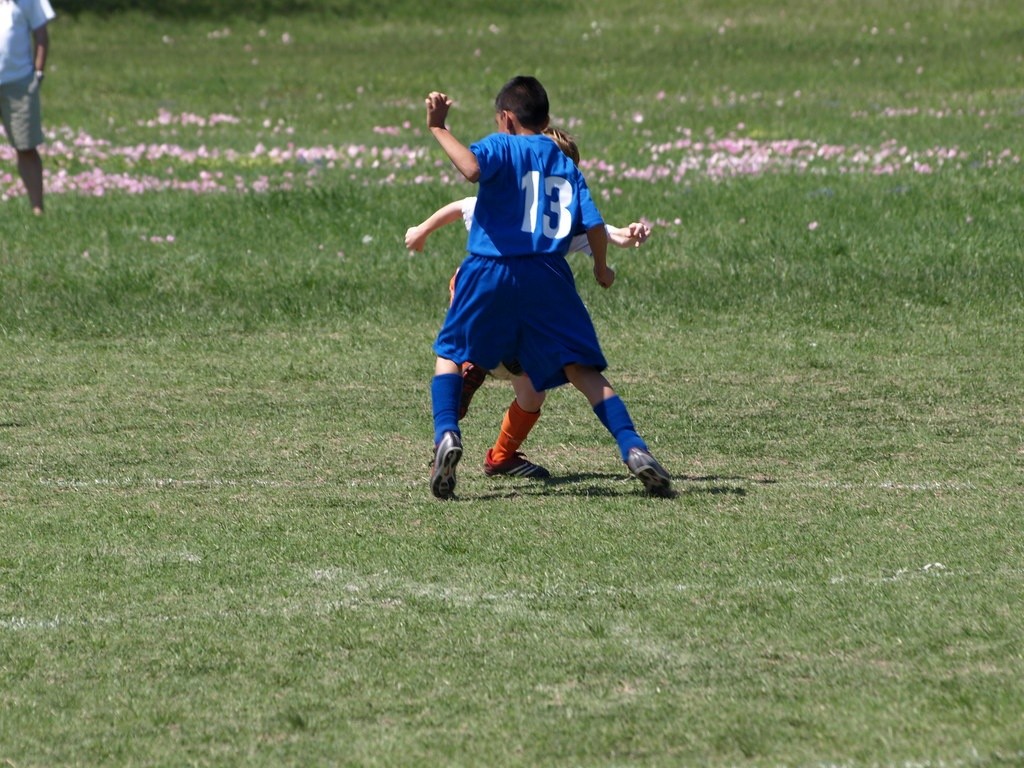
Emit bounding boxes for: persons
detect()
[422,74,674,495]
[0,0,50,214]
[403,124,650,480]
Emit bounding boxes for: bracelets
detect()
[36,70,44,80]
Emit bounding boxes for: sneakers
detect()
[429,429,463,499]
[628,448,670,489]
[485,448,551,481]
